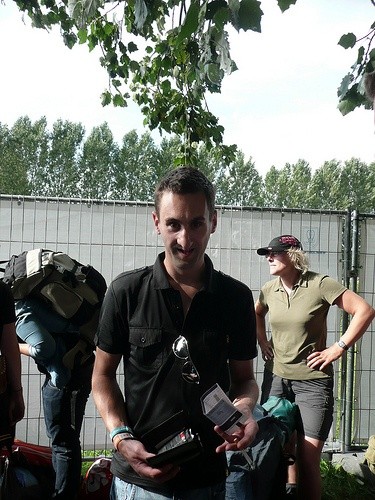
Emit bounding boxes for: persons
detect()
[91,167,259,500]
[255,235,375,500]
[0,277,95,500]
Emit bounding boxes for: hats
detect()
[257,235,303,255]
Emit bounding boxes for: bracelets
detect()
[109,425,132,443]
[337,340,348,351]
[114,434,136,452]
[10,386,22,393]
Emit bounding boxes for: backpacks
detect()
[224,403,296,500]
[261,396,297,435]
[0,249,107,345]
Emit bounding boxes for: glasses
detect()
[265,252,287,258]
[172,335,200,385]
